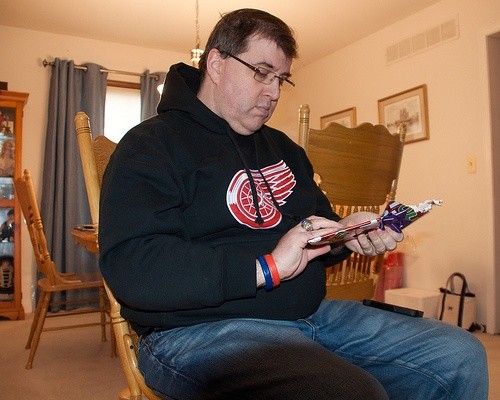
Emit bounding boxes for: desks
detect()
[70,229,98,253]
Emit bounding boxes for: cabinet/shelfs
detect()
[0,91,25,320]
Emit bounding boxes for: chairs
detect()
[12,108,163,400]
[298,104,408,304]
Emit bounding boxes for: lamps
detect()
[157,1,207,95]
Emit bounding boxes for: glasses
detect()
[219,50,295,96]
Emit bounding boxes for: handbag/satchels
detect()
[437,272,477,334]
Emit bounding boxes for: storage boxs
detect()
[381,287,441,318]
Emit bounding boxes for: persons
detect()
[98,8,488,400]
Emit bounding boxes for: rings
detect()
[300,218,314,232]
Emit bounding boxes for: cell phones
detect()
[363,299,424,318]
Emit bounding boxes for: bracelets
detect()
[257,254,280,290]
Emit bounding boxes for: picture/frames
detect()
[376,83,430,145]
[319,106,357,131]
